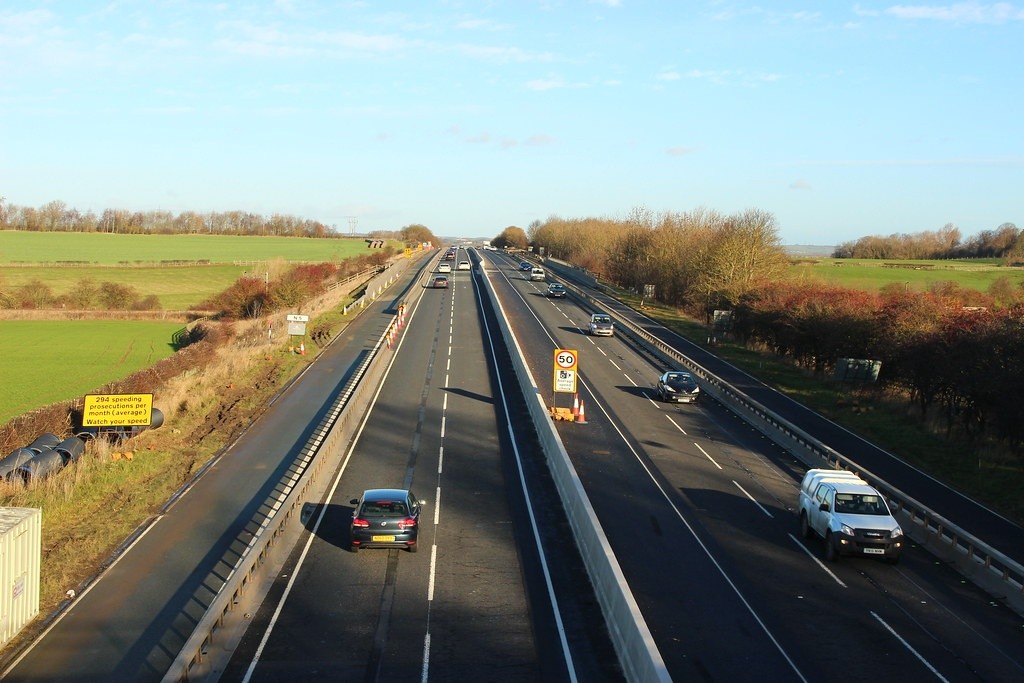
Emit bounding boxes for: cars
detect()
[432,276,449,289]
[438,263,452,273]
[457,261,472,271]
[446,244,464,260]
[588,313,616,337]
[531,267,546,282]
[655,371,700,404]
[348,488,426,553]
[546,282,567,299]
[518,261,532,272]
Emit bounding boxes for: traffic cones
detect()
[571,393,580,416]
[300,341,306,356]
[574,400,589,425]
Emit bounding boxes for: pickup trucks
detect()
[797,469,905,565]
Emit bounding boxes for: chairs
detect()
[393,502,405,512]
[366,503,381,512]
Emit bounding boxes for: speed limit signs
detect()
[556,351,576,368]
